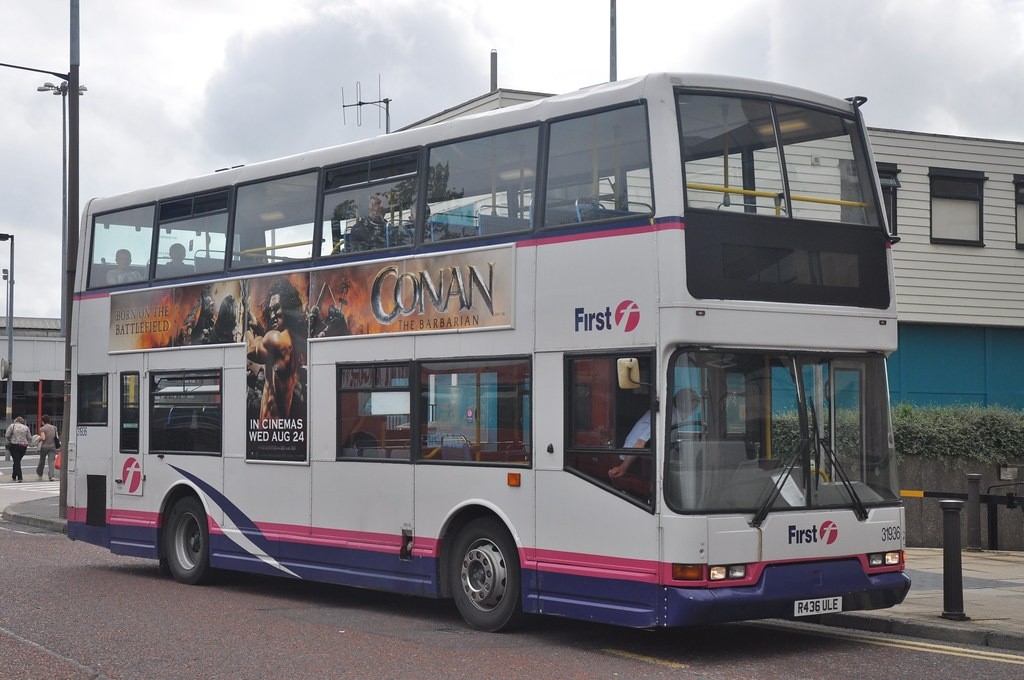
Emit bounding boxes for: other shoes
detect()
[49,477,54,481]
[12,476,16,480]
[36,470,43,476]
[18,478,23,481]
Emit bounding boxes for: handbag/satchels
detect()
[53,427,61,449]
[5,437,12,450]
[55,452,61,469]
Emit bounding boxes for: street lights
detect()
[38,82,87,336]
[0,233,15,460]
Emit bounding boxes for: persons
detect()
[107,249,143,285]
[162,244,192,278]
[178,280,349,457]
[350,205,391,252]
[608,388,700,503]
[36,415,58,480]
[5,417,32,482]
[394,203,450,247]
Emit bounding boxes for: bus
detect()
[61,74,911,634]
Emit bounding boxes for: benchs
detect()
[346,433,527,464]
[93,248,257,287]
[343,201,653,254]
